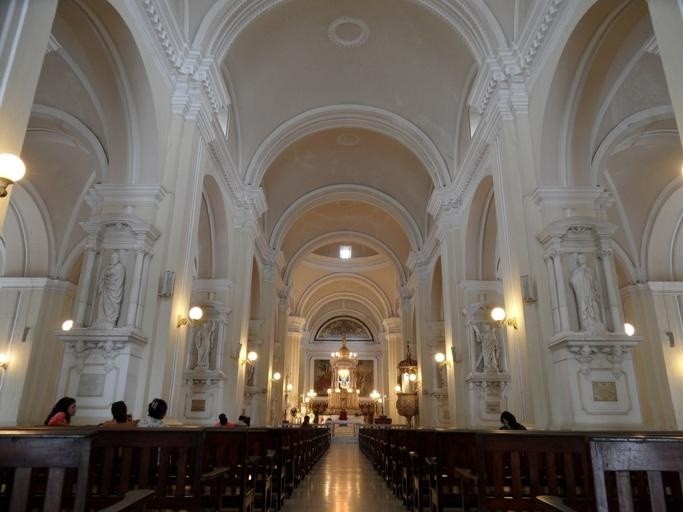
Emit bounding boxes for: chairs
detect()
[358,427,683,512]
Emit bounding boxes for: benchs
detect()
[1,423,330,511]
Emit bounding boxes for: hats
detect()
[151,399,167,413]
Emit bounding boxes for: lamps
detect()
[410,374,420,384]
[433,351,451,370]
[284,384,293,393]
[1,153,28,197]
[242,351,259,368]
[490,307,518,330]
[177,304,204,330]
[270,372,281,383]
[328,332,361,370]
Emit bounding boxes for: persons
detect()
[300,416,312,427]
[215,413,248,427]
[136,399,173,467]
[91,252,125,329]
[474,324,501,373]
[498,411,527,430]
[568,253,601,333]
[42,396,76,425]
[194,320,214,368]
[97,399,136,455]
